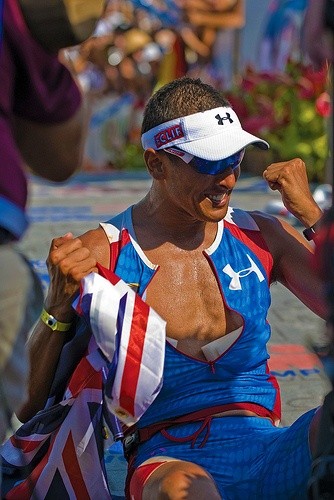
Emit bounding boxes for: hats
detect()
[141,105,270,162]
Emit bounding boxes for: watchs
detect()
[40,308,71,332]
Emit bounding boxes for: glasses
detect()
[161,147,248,175]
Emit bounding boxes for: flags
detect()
[0,263,168,500]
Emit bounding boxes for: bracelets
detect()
[302,208,332,241]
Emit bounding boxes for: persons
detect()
[18,77,334,500]
[0,0,85,247]
